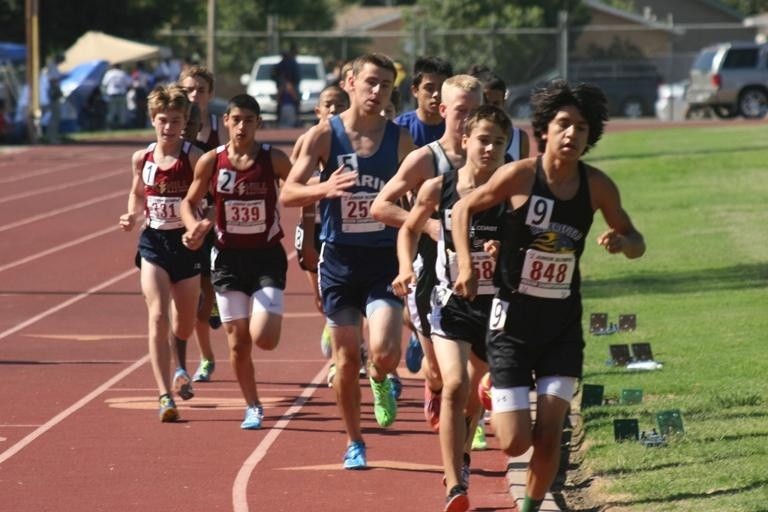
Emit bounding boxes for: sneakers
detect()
[443,452,470,511]
[342,440,367,471]
[208,296,221,330]
[472,420,490,451]
[158,358,263,429]
[321,317,442,432]
[479,373,491,413]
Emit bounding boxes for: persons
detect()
[42,54,68,145]
[103,61,154,130]
[273,46,303,129]
[370,74,483,429]
[118,82,205,422]
[280,54,488,468]
[180,94,294,430]
[390,105,513,512]
[175,65,221,381]
[450,82,645,512]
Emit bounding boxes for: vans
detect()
[684,42,767,119]
[497,62,658,121]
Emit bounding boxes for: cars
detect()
[655,72,728,122]
[134,72,234,128]
[240,54,338,131]
